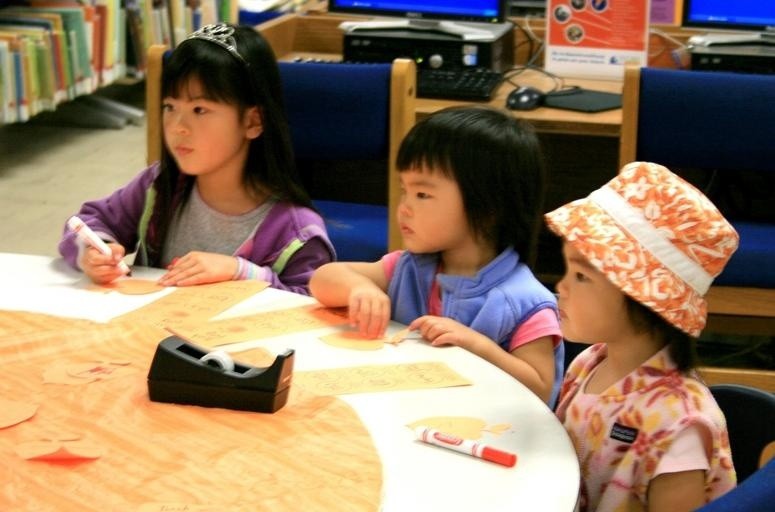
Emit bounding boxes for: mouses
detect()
[506,86,545,109]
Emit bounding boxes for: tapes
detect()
[199,325,360,372]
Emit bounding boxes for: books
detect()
[0,0,235,124]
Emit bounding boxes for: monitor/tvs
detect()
[327,0,506,40]
[681,0,775,47]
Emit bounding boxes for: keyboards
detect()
[289,55,503,102]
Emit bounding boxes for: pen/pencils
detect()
[65,215,132,278]
[416,426,518,468]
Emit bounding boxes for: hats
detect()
[544,160,741,340]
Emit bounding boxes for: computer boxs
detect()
[341,27,516,74]
[690,41,775,76]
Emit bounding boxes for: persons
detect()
[59,22,334,296]
[309,106,566,408]
[540,160,740,512]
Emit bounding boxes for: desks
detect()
[0,252,581,512]
